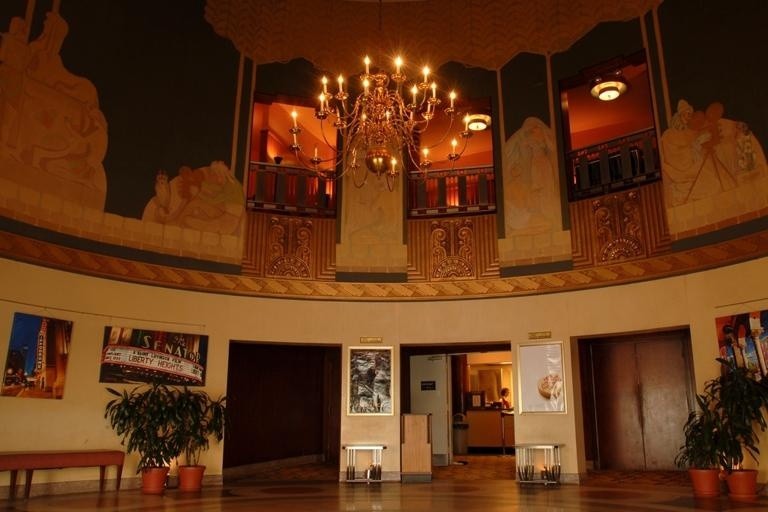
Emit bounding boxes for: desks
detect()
[0,449,124,502]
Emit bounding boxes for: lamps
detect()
[460,110,492,132]
[587,69,627,101]
[289,0,471,193]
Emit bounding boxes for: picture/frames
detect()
[516,339,567,416]
[346,345,394,418]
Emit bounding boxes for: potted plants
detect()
[103,378,228,496]
[672,357,767,503]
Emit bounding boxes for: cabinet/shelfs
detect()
[401,413,432,483]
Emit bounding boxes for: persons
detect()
[499,388,512,409]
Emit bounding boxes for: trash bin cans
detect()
[453,422,469,455]
